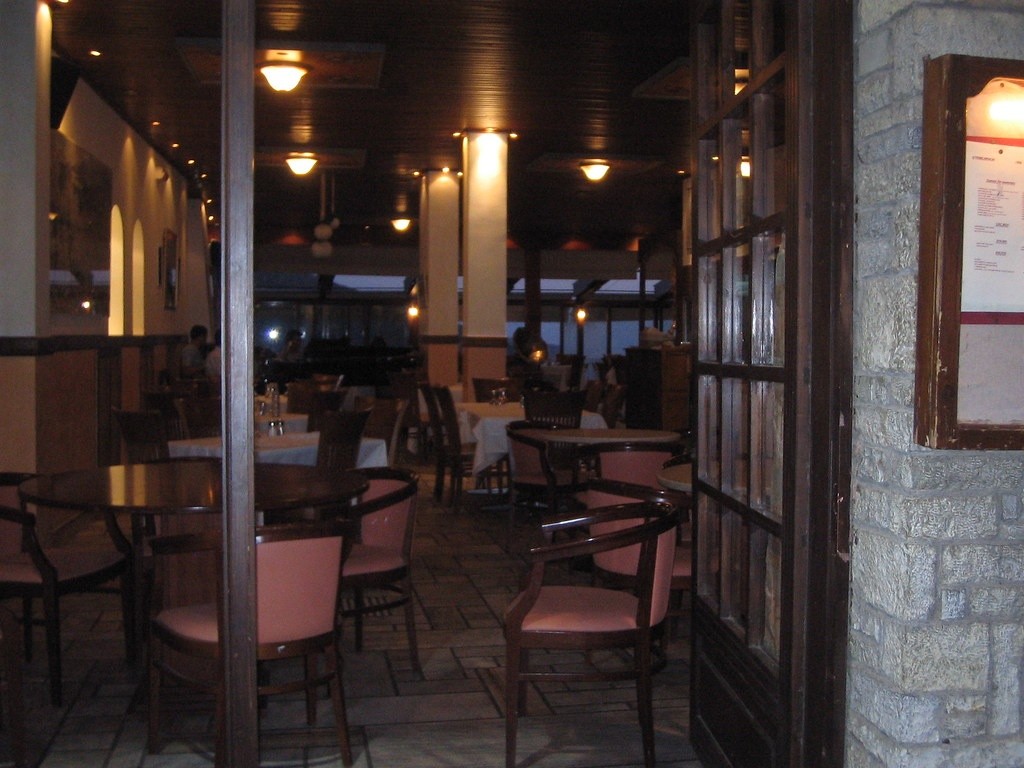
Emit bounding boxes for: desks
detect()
[167,432,389,527]
[250,393,288,414]
[253,414,309,432]
[456,402,609,514]
[15,460,369,711]
[542,427,682,570]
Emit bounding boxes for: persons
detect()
[179,324,304,384]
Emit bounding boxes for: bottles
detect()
[265,387,274,397]
[269,421,277,437]
[489,390,509,405]
[277,421,285,435]
[259,402,267,416]
[273,388,280,418]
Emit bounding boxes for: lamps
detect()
[256,60,315,93]
[390,216,411,232]
[408,303,419,318]
[577,159,613,182]
[282,151,320,175]
[576,305,586,321]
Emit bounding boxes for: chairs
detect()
[0,339,695,768]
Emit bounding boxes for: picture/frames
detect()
[161,225,179,314]
